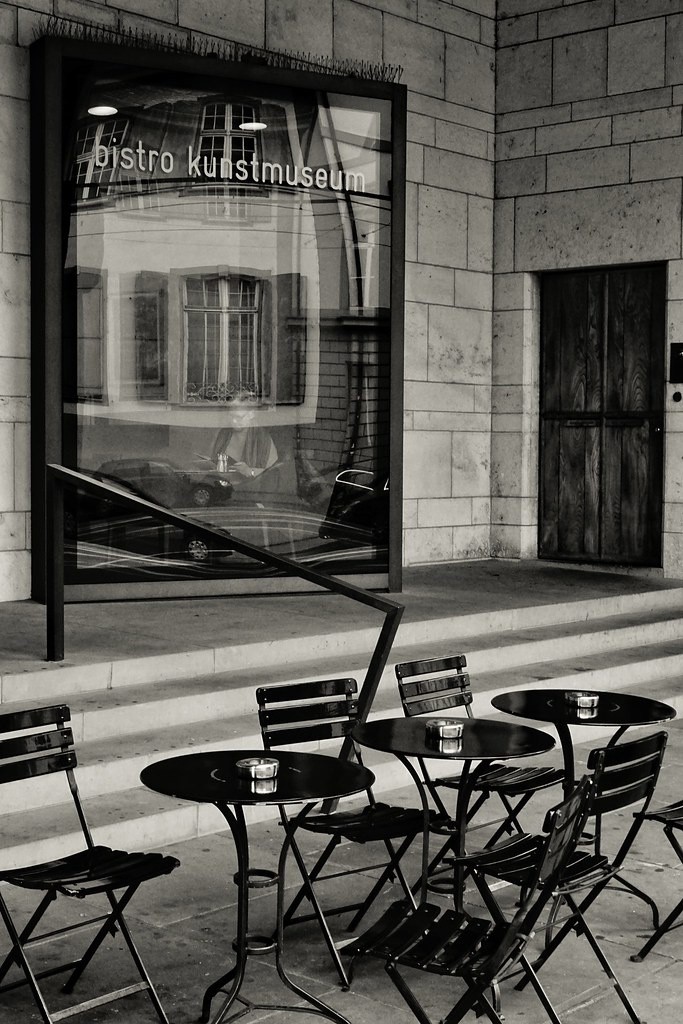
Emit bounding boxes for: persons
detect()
[188,391,278,485]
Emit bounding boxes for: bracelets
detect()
[250,468,255,477]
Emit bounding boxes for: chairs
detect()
[630,800,683,962]
[396,655,567,901]
[341,775,590,1024]
[441,730,668,1024]
[255,678,451,992]
[0,705,179,1024]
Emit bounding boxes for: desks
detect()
[490,689,677,928]
[140,750,376,1024]
[352,717,556,1021]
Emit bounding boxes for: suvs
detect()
[92,457,236,512]
